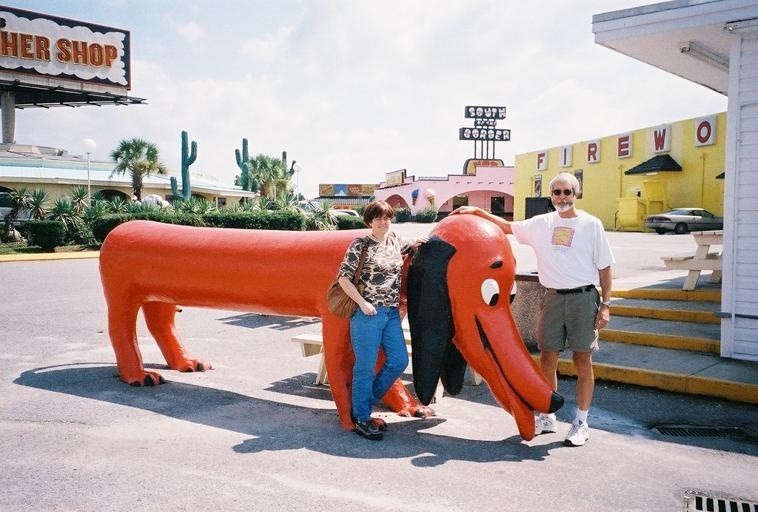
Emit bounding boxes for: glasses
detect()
[552,190,571,195]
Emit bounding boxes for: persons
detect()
[338,198,430,440]
[448,172,616,450]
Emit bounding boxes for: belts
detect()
[547,284,595,294]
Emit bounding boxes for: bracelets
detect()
[601,301,611,308]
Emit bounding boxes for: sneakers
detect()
[355,420,383,440]
[534,413,557,435]
[564,417,589,446]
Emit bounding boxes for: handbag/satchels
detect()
[327,238,368,319]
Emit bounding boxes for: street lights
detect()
[295,166,301,200]
[85,151,93,206]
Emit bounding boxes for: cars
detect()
[645,208,722,235]
[299,201,360,217]
[0,192,34,239]
